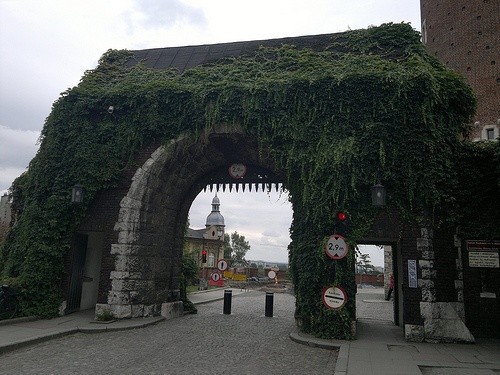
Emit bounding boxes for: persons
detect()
[385,269,394,301]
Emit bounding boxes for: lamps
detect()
[370,175,387,207]
[71,177,84,203]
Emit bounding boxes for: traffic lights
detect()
[335,209,348,222]
[202,250,207,263]
